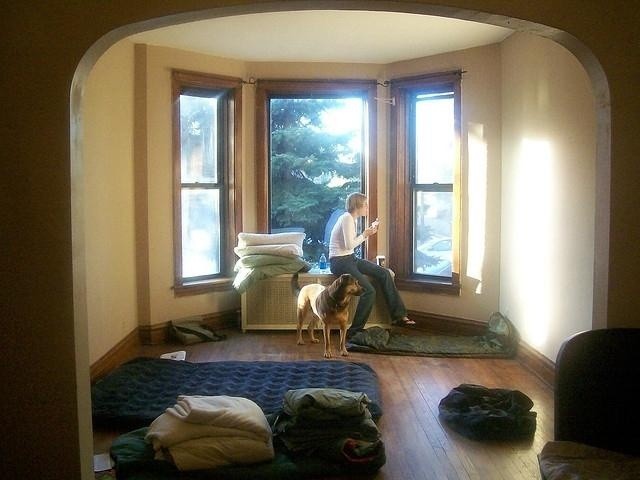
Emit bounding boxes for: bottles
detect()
[319,252,326,269]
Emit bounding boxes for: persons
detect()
[328,192,416,340]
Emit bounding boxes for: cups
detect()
[375,255,386,269]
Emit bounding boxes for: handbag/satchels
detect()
[168,319,214,345]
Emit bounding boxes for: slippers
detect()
[401,317,416,326]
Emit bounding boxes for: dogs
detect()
[291,273,367,358]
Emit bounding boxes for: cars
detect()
[416,237,453,262]
